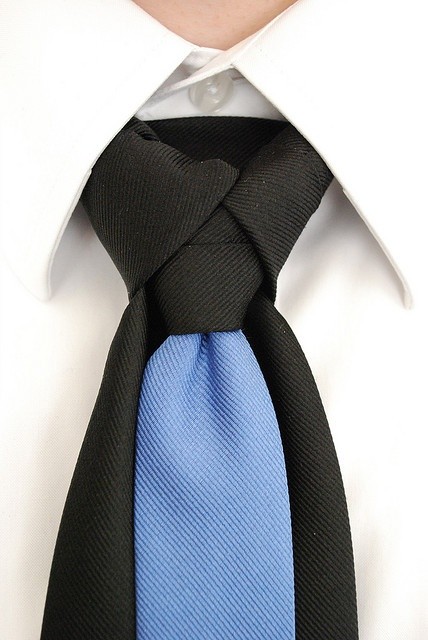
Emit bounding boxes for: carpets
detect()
[41,117,358,635]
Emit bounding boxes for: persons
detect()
[1,0,427,640]
